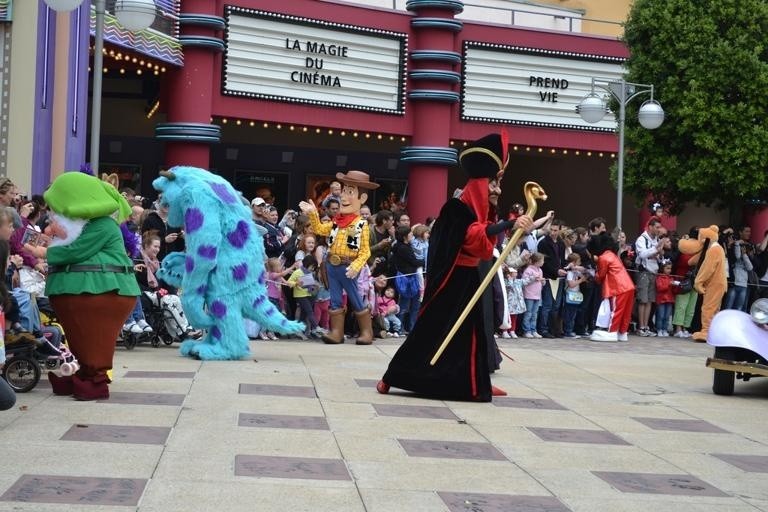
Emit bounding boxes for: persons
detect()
[4,129,767,410]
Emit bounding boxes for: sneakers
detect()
[510,331,518,338]
[266,331,279,340]
[260,331,269,340]
[522,333,534,337]
[532,332,543,338]
[673,331,686,338]
[502,331,511,339]
[394,332,399,338]
[646,330,657,337]
[126,322,143,333]
[564,332,581,339]
[138,319,154,332]
[636,329,649,337]
[387,332,393,337]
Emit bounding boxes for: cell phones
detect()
[176,231,183,236]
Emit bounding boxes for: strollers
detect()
[11,289,81,373]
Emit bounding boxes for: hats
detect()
[251,197,265,206]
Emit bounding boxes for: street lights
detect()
[44,1,152,181]
[577,75,664,232]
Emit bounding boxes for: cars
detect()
[706,291,767,395]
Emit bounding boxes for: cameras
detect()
[726,231,738,244]
[134,193,149,205]
[17,192,29,203]
[377,255,388,264]
[385,233,394,246]
[659,232,674,253]
[738,239,758,259]
[290,210,300,221]
[549,211,558,220]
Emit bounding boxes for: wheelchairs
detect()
[3,327,51,391]
[114,311,162,347]
[141,285,204,344]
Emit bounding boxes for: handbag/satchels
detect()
[566,290,584,304]
[672,278,692,295]
[396,271,419,299]
